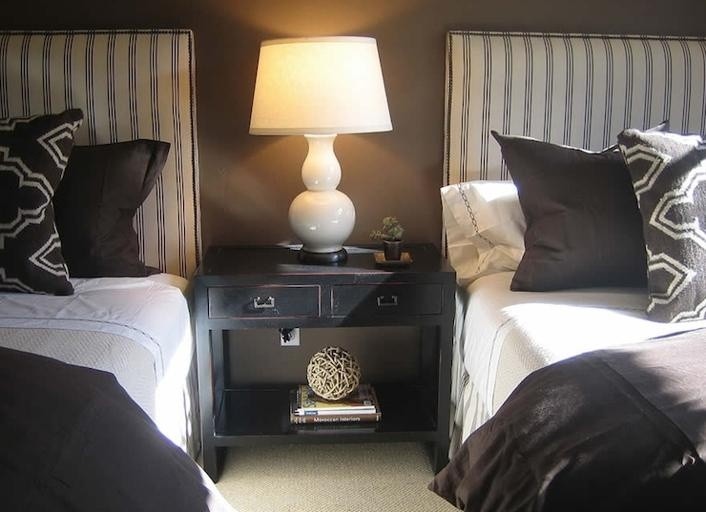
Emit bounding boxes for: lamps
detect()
[247,34,394,267]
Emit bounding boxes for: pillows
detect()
[617,128,705,322]
[438,182,529,285]
[490,131,649,291]
[0,108,172,298]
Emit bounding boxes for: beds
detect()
[439,26,705,509]
[0,26,206,512]
[439,26,706,509]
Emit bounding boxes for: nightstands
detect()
[195,244,455,483]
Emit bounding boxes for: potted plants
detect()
[370,216,406,261]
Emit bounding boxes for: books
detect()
[288,382,382,424]
